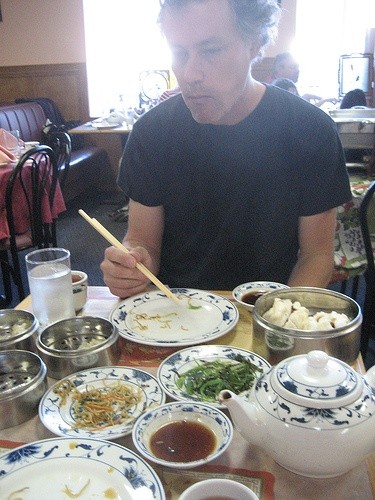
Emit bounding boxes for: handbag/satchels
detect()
[42,119,83,154]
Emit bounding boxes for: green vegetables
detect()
[176,357,264,401]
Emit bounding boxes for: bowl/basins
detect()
[132,402,233,469]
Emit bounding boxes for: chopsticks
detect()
[78,209,180,302]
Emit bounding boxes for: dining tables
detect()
[0,146,67,302]
[0,284,375,500]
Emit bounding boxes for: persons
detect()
[267,51,303,97]
[99,0,354,297]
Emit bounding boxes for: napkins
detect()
[0,128,18,150]
[0,145,18,164]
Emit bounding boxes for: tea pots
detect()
[219,350,375,479]
[105,111,124,124]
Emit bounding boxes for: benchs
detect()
[0,102,108,225]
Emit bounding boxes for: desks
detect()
[68,119,134,194]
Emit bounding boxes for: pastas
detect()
[54,377,144,431]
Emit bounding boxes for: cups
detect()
[71,270,89,312]
[25,248,76,329]
[125,110,134,129]
[178,478,259,500]
[25,142,39,152]
[3,130,19,155]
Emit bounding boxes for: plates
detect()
[109,288,239,347]
[0,436,166,500]
[157,345,272,409]
[91,122,116,128]
[232,281,290,313]
[38,366,166,440]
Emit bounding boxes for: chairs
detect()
[0,127,72,303]
[341,182,375,358]
